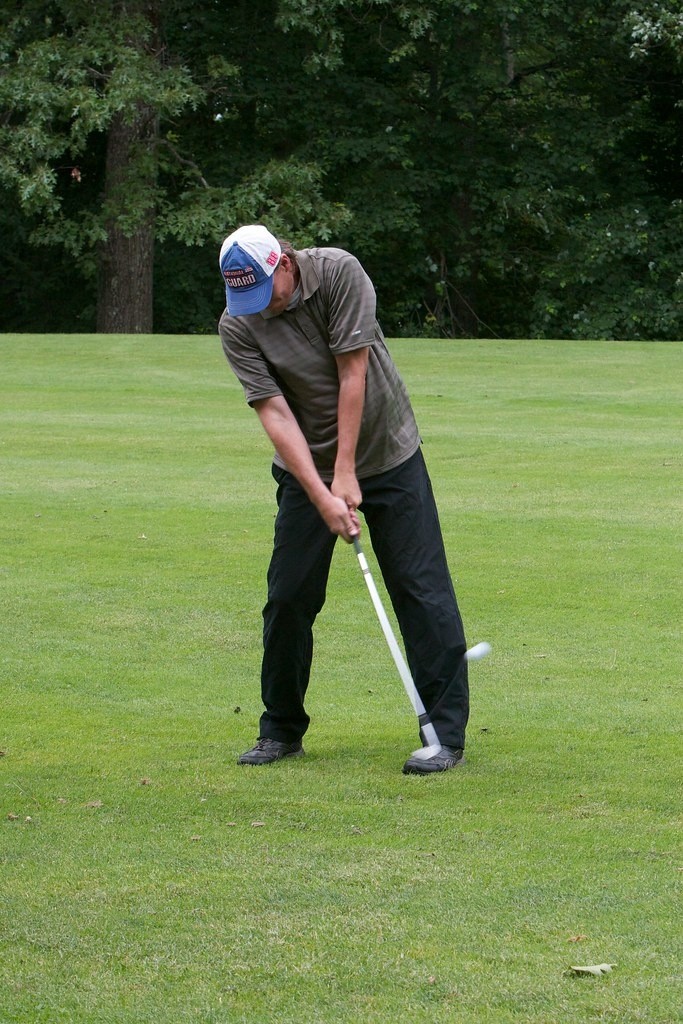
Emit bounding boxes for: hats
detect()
[219,224,280,316]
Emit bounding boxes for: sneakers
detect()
[402,745,465,775]
[237,738,305,766]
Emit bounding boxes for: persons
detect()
[217,223,469,773]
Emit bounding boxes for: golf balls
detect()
[463,641,492,662]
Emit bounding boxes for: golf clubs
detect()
[349,507,442,761]
